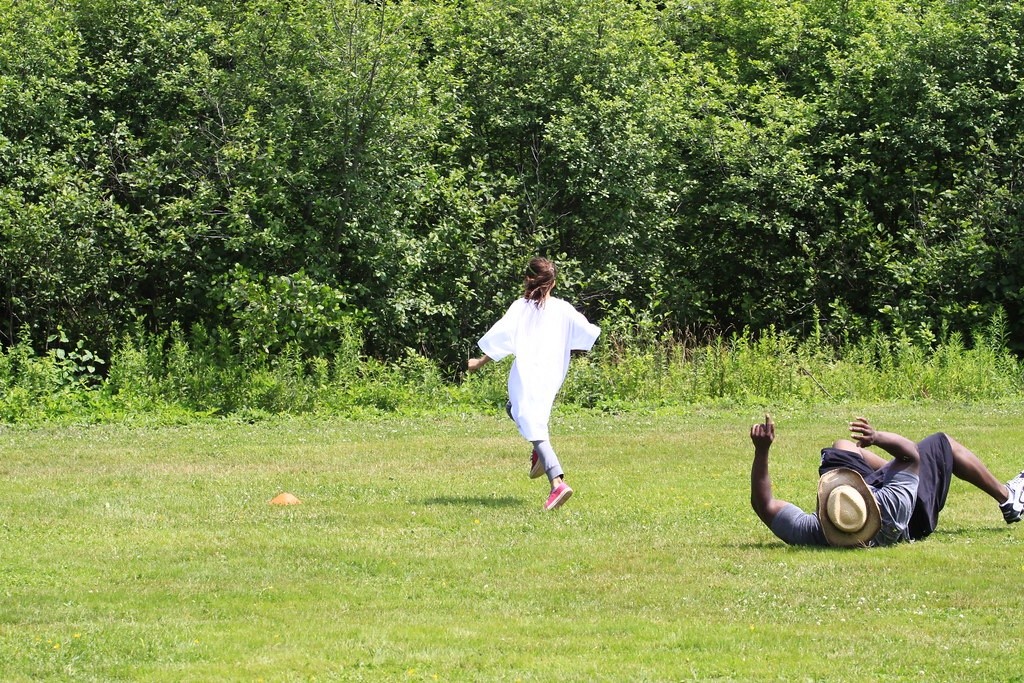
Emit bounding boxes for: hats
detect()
[816,467,882,550]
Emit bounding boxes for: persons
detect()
[466,259,602,510]
[748,414,1024,549]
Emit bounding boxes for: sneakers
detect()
[543,483,573,512]
[1000,469,1024,524]
[529,449,547,478]
